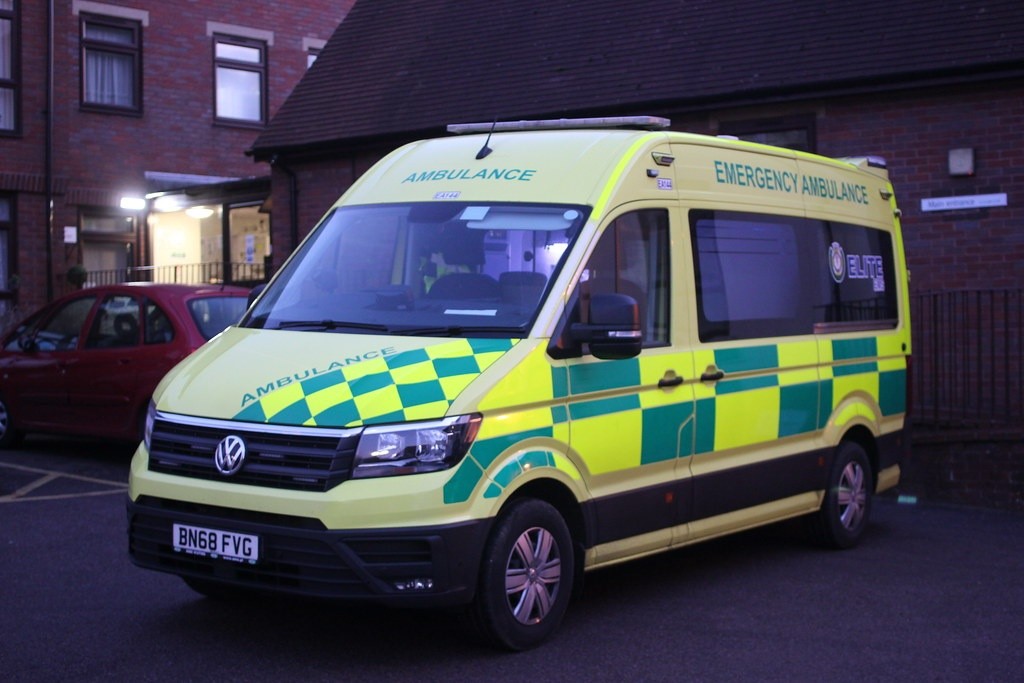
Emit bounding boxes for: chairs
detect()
[99,313,139,347]
[499,272,547,305]
[427,272,500,301]
[579,278,649,337]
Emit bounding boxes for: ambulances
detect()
[120,111,915,655]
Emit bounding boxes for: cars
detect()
[0,279,253,449]
